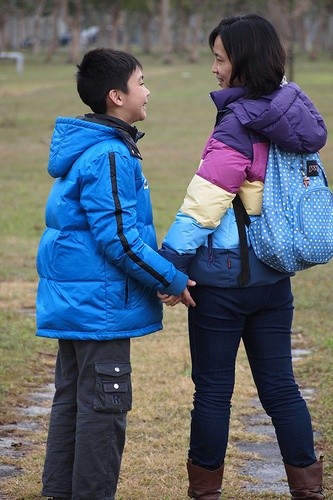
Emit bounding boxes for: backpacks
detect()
[216,109,332,274]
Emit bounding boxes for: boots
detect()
[282,456,325,500]
[186,462,223,500]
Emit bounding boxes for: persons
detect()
[35,49,196,500]
[157,15,328,500]
